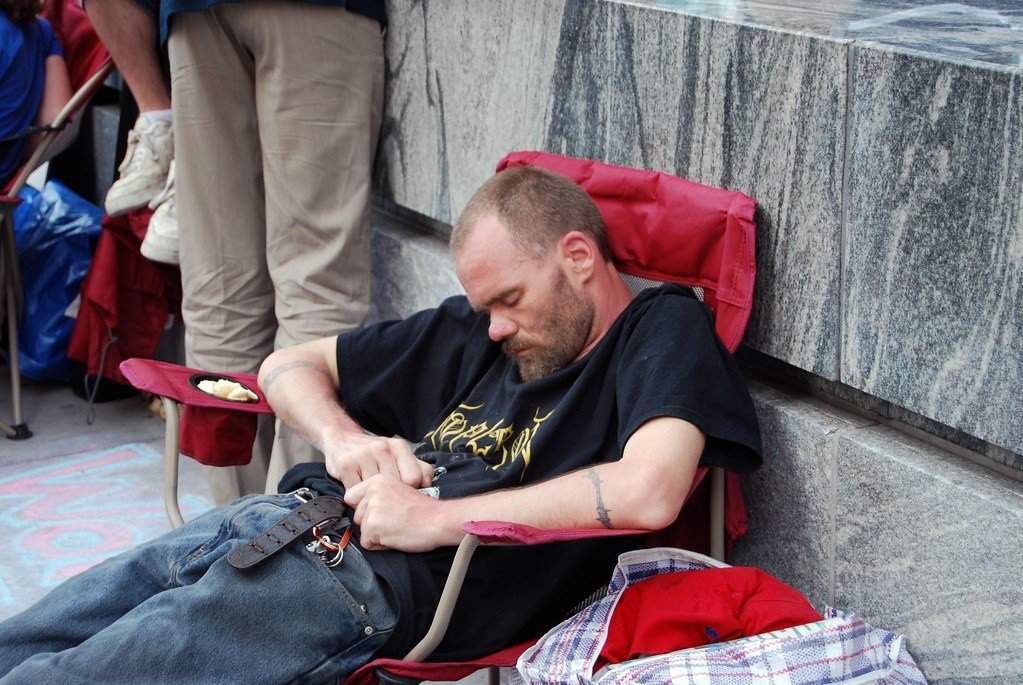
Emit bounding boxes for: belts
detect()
[227,485,362,571]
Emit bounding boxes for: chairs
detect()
[119,151,758,685]
[0,1,117,441]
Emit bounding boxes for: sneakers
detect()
[103,113,177,216]
[140,156,179,264]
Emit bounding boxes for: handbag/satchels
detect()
[516,548,927,685]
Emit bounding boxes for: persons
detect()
[0,168,764,685]
[0,0,389,510]
